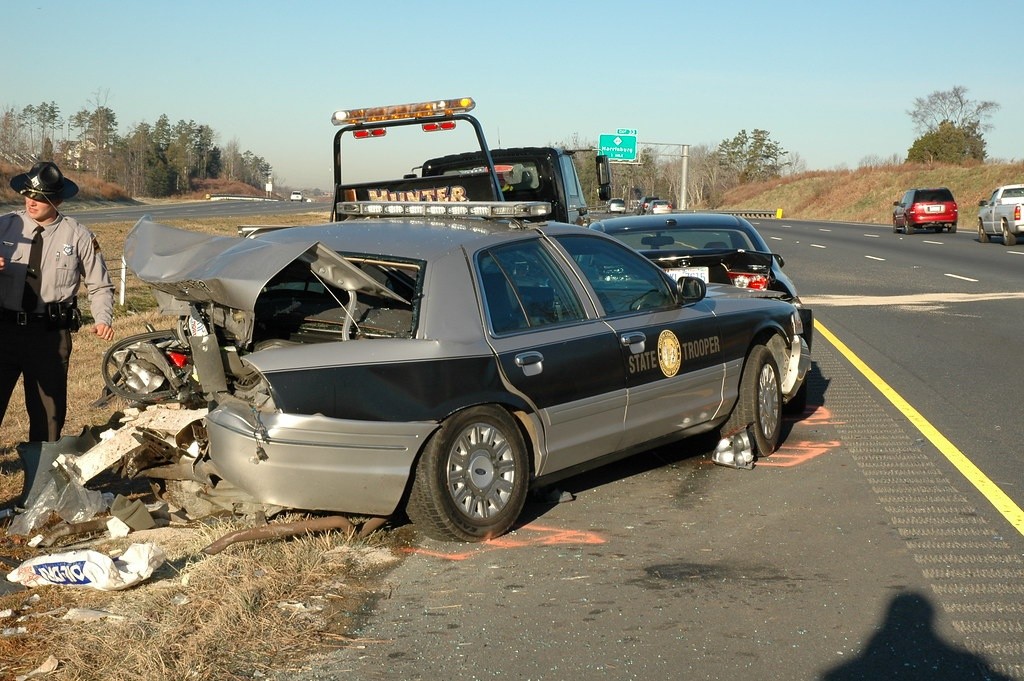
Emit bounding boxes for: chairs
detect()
[482,269,551,330]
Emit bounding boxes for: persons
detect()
[0,162,116,445]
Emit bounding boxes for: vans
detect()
[636,196,659,214]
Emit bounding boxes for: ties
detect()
[21,226,45,314]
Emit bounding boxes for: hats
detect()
[10,162,78,202]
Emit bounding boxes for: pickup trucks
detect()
[977,183,1024,247]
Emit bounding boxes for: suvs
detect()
[891,187,958,235]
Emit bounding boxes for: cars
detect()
[575,210,808,364]
[96,97,815,543]
[290,191,303,202]
[605,198,626,214]
[645,199,674,217]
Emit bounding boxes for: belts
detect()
[0,307,49,326]
